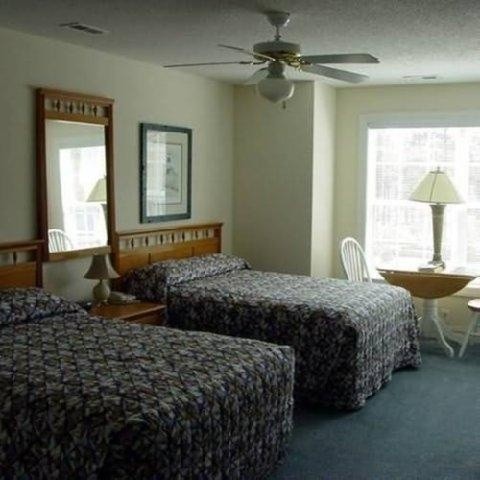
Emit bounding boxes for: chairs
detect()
[458,298,480,359]
[48,228,73,254]
[340,237,372,284]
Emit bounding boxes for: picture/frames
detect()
[139,122,193,222]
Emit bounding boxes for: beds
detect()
[115,220,422,412]
[0,240,295,480]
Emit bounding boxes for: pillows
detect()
[137,251,251,287]
[0,284,77,326]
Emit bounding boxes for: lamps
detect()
[86,178,108,224]
[258,62,294,105]
[409,165,465,269]
[85,255,121,306]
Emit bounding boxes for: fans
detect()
[163,14,380,85]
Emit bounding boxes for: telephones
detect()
[110,292,136,302]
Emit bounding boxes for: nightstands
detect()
[86,299,167,327]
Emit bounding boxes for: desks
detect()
[378,261,480,358]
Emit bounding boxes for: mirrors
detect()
[33,87,115,262]
